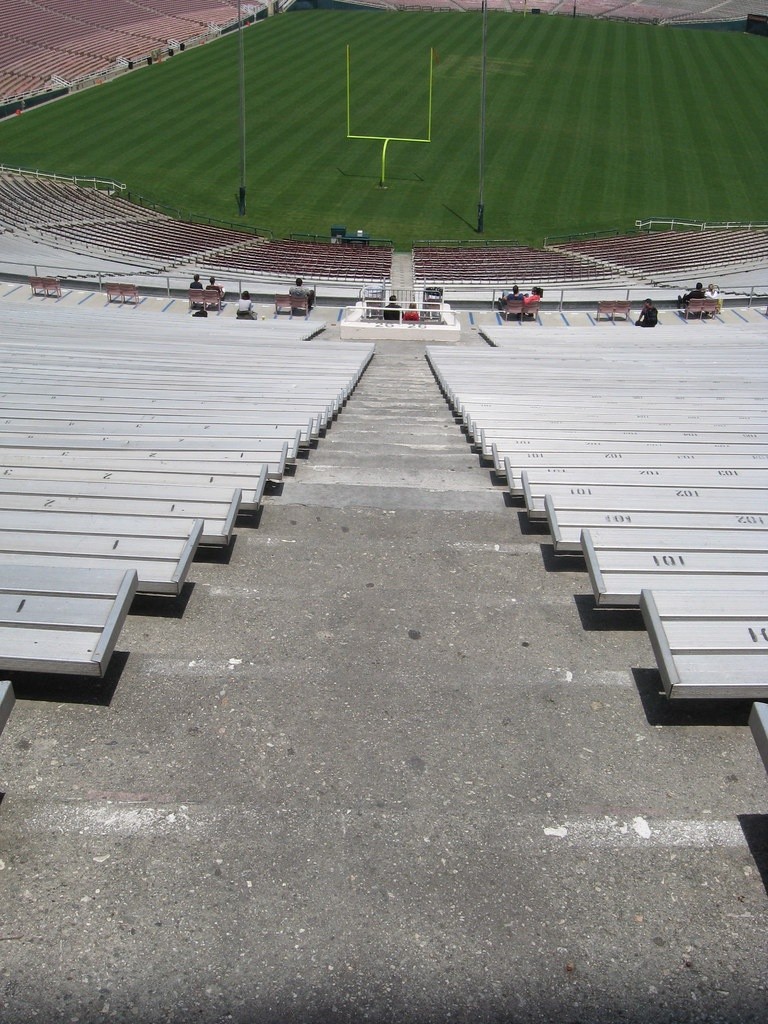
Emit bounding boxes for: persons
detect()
[190,274,202,289]
[502,285,524,306]
[206,276,224,297]
[237,291,254,315]
[289,278,315,310]
[384,296,404,320]
[684,282,705,307]
[635,299,658,327]
[403,303,419,321]
[705,284,718,300]
[524,287,542,317]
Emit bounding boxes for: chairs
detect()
[684,298,705,319]
[30,277,47,296]
[119,283,139,305]
[365,286,382,315]
[521,301,540,323]
[106,282,124,303]
[502,299,525,324]
[185,289,207,309]
[275,293,293,319]
[614,299,631,322]
[42,277,62,298]
[700,298,718,320]
[290,294,311,320]
[596,300,616,322]
[423,288,443,316]
[203,290,222,311]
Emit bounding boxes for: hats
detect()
[643,298,652,303]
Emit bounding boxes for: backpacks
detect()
[645,307,658,325]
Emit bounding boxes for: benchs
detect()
[0,166,768,774]
